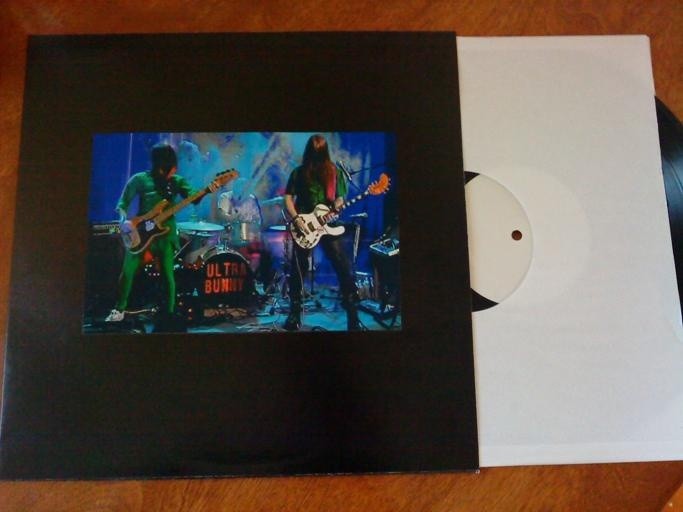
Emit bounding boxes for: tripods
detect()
[261,207,322,315]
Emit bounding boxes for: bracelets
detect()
[293,215,300,220]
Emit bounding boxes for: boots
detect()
[283,303,300,330]
[346,304,361,331]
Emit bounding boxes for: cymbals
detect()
[270,225,290,231]
[176,221,225,238]
[263,196,284,206]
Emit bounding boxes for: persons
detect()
[104,140,218,323]
[281,135,360,332]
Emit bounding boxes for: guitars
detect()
[118,168,239,256]
[289,173,391,250]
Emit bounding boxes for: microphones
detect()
[336,160,352,181]
[349,212,368,219]
[250,193,257,200]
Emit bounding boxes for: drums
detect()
[228,220,258,248]
[192,244,255,309]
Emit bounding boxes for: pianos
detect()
[90,223,121,240]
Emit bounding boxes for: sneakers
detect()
[105,309,124,321]
